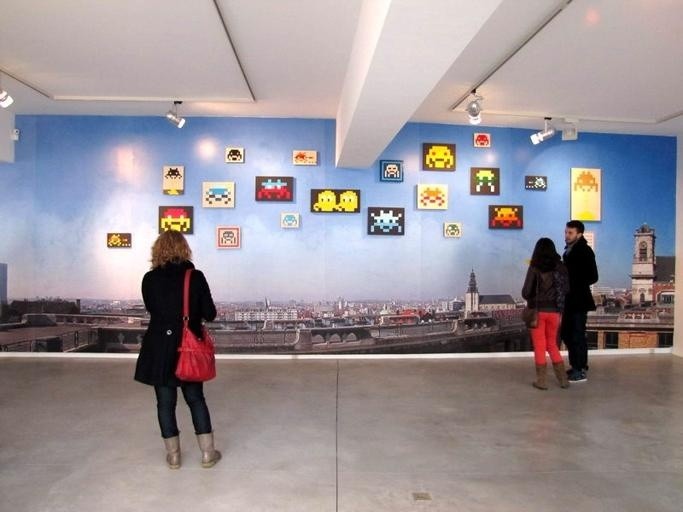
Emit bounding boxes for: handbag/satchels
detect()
[522,306,538,328]
[176,328,216,381]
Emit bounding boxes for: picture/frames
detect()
[379,159,404,182]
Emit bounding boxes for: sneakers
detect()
[567,364,588,383]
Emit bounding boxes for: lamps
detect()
[529,116,557,146]
[164,101,186,130]
[463,94,486,125]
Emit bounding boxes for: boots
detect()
[553,360,569,387]
[164,436,181,469]
[196,432,221,468]
[533,363,547,389]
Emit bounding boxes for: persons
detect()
[560,221,598,383]
[134,230,222,469]
[522,238,570,390]
